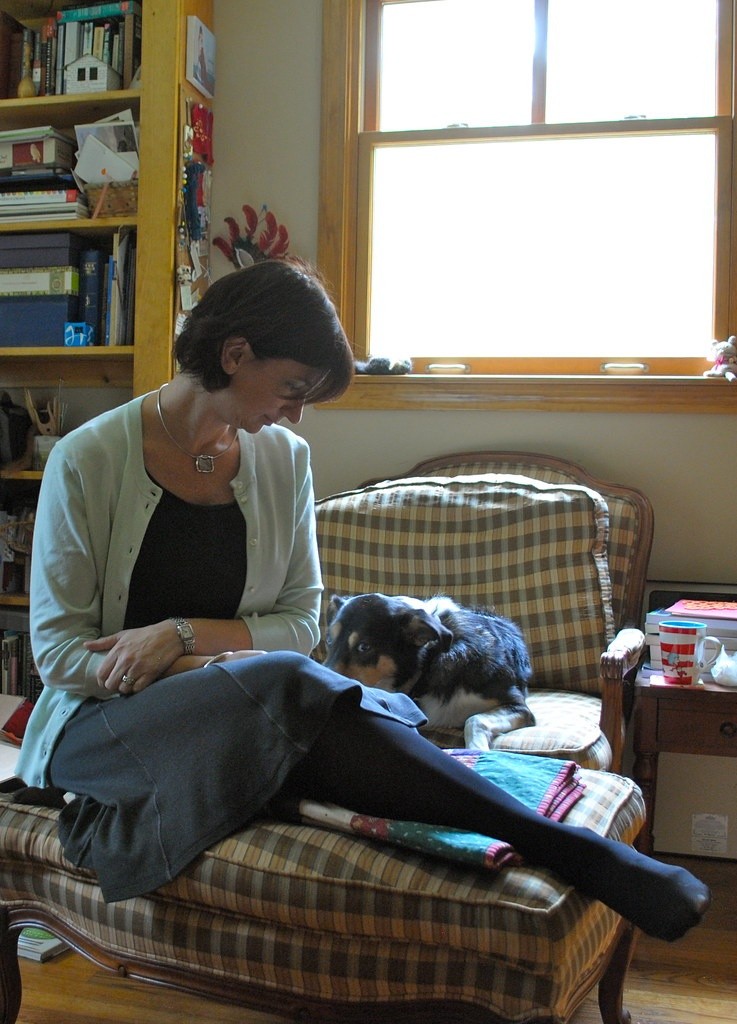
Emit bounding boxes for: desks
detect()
[633,662,737,859]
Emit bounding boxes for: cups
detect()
[659,621,721,683]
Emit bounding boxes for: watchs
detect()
[169,617,195,656]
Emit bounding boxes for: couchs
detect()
[0,453,654,1024]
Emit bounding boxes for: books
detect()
[0,124,89,223]
[17,927,70,964]
[645,599,737,671]
[77,232,137,346]
[0,629,45,707]
[0,0,142,102]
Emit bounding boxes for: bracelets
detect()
[202,651,232,668]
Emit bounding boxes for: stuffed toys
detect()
[703,335,737,383]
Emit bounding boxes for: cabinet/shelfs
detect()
[0,0,217,706]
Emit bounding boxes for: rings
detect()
[122,675,136,685]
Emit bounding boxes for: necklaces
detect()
[157,383,238,473]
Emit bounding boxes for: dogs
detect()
[321,593,536,750]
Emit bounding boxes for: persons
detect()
[15,258,712,943]
